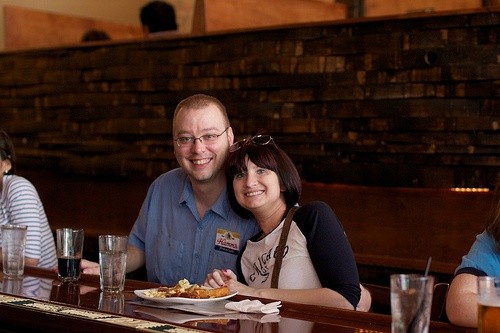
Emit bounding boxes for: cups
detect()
[2,277,23,295]
[55,228,84,283]
[476,276,500,333]
[390,274,434,333]
[50,282,80,306]
[0,225,28,277]
[98,292,124,314]
[98,235,127,294]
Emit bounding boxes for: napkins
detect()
[225,299,282,315]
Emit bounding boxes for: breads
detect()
[181,285,229,298]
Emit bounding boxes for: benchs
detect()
[29,176,500,321]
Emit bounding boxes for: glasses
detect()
[173,127,229,145]
[229,135,277,153]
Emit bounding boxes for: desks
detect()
[1,266,480,333]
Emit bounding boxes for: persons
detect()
[445,202,500,328]
[202,135,362,311]
[0,130,59,270]
[52,95,372,313]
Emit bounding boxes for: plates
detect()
[133,287,239,304]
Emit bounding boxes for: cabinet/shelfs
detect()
[1,8,500,190]
